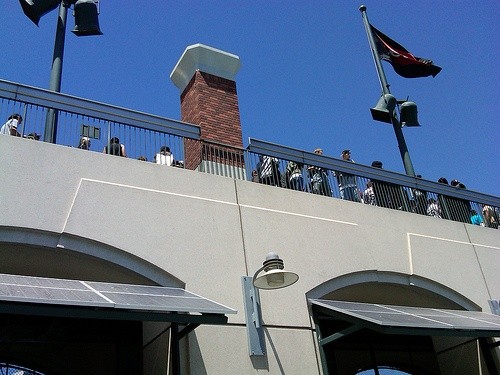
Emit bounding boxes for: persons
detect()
[103,137,126,157]
[0,113,35,140]
[255,149,500,229]
[154,145,184,166]
[77,137,92,151]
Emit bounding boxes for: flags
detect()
[368,23,442,79]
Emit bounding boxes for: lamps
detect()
[242,252,299,358]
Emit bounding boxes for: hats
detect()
[341,150,351,155]
[371,161,383,168]
